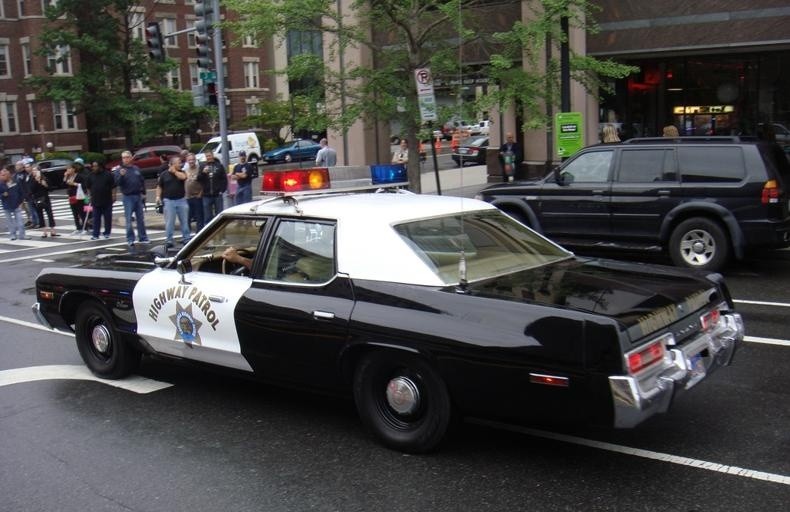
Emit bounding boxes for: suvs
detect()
[472,131,790,275]
[105,145,183,175]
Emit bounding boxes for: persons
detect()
[499,132,526,176]
[661,124,679,136]
[601,124,620,142]
[314,137,337,166]
[1,147,253,247]
[392,139,410,167]
[223,222,266,272]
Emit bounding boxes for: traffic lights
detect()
[146,22,167,63]
[196,34,215,72]
[194,2,213,33]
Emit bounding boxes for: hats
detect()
[74,157,84,166]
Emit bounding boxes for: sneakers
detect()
[10,221,150,246]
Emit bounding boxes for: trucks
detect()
[184,132,262,167]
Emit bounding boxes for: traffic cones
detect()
[435,134,442,149]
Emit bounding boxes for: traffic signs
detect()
[198,71,217,80]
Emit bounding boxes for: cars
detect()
[31,157,92,188]
[451,135,489,165]
[263,138,320,162]
[389,119,490,145]
[736,123,790,143]
[30,159,744,454]
